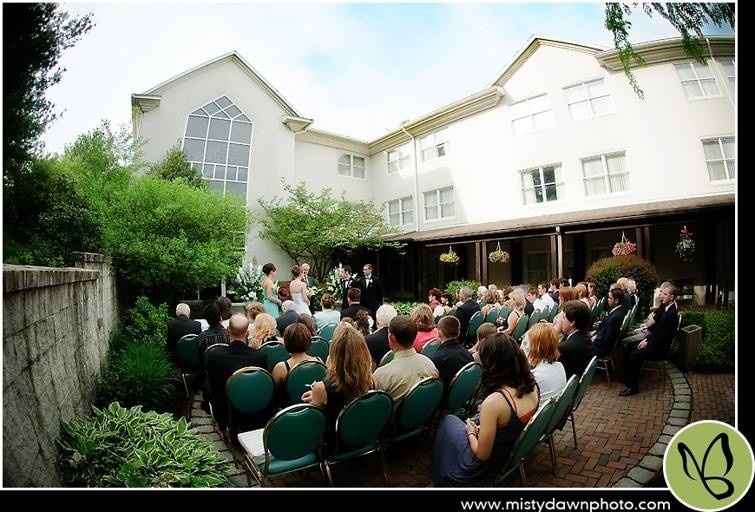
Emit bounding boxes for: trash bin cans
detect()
[677,324,702,372]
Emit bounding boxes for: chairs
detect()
[178,288,681,487]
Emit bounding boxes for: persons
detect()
[168,263,679,487]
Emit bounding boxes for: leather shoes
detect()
[618,387,639,396]
